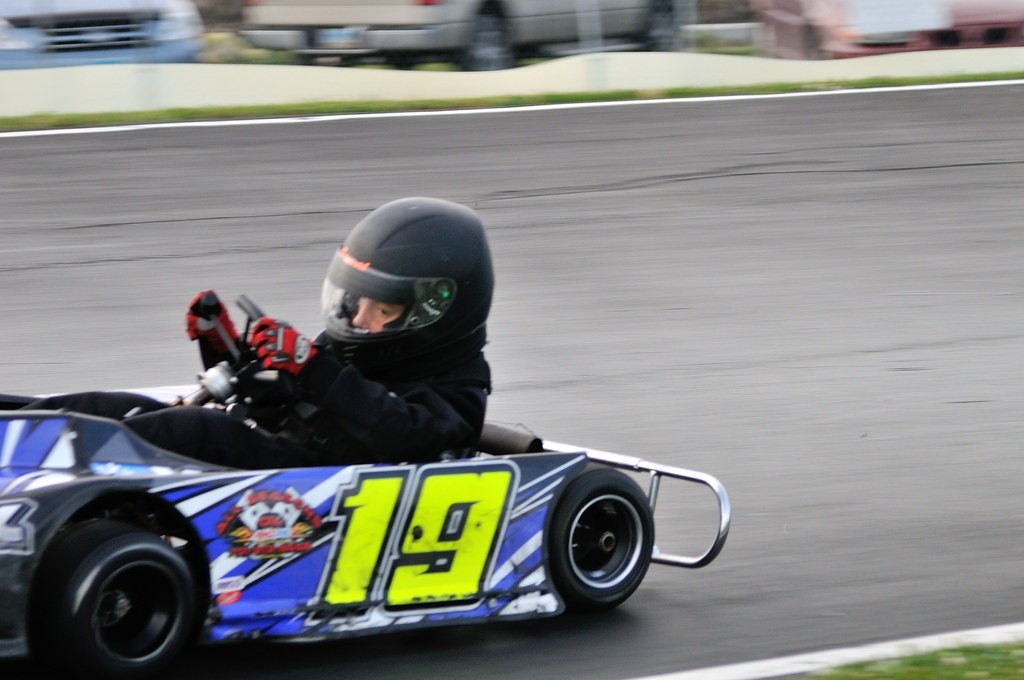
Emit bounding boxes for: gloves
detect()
[185,289,239,353]
[246,317,319,375]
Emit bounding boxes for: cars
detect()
[0,0,210,71]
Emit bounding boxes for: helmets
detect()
[321,197,494,382]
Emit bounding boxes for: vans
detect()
[236,1,698,76]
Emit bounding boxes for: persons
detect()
[13,195,494,472]
